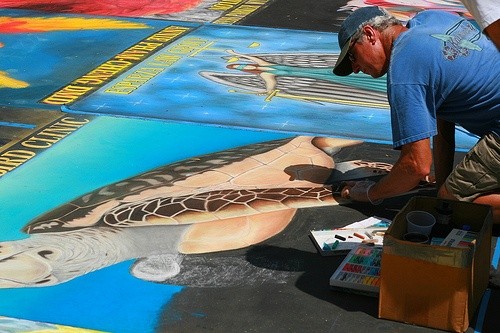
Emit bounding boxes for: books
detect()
[310,228,390,257]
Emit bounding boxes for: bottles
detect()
[435,201,453,231]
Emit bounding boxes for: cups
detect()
[403,232,429,245]
[406,211,436,237]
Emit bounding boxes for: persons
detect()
[458,0,500,49]
[334,5,500,223]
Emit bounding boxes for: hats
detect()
[332,6,394,78]
